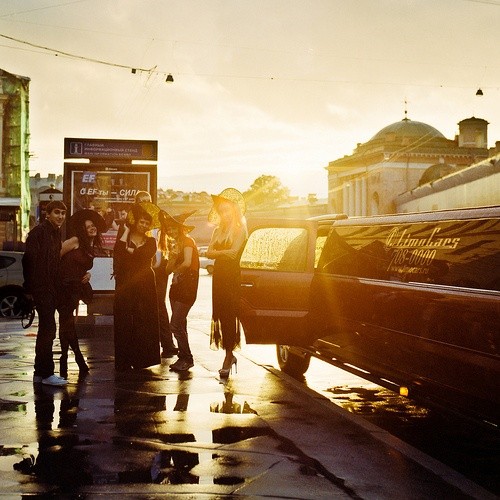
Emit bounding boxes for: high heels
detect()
[219,356,237,377]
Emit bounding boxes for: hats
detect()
[71,209,108,233]
[206,185,246,225]
[158,209,195,239]
[126,201,162,231]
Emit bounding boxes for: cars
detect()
[236,211,500,431]
[0,250,26,318]
[199,258,216,274]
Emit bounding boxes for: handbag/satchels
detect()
[81,282,94,306]
[12,292,36,330]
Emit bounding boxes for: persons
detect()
[113,201,162,372]
[116,206,130,240]
[158,203,200,372]
[135,191,179,358]
[206,188,248,379]
[57,209,107,377]
[22,199,68,386]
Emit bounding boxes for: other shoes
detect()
[59,356,68,380]
[74,354,89,372]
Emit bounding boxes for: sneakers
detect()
[42,375,68,385]
[169,358,194,371]
[161,348,178,358]
[32,372,64,385]
